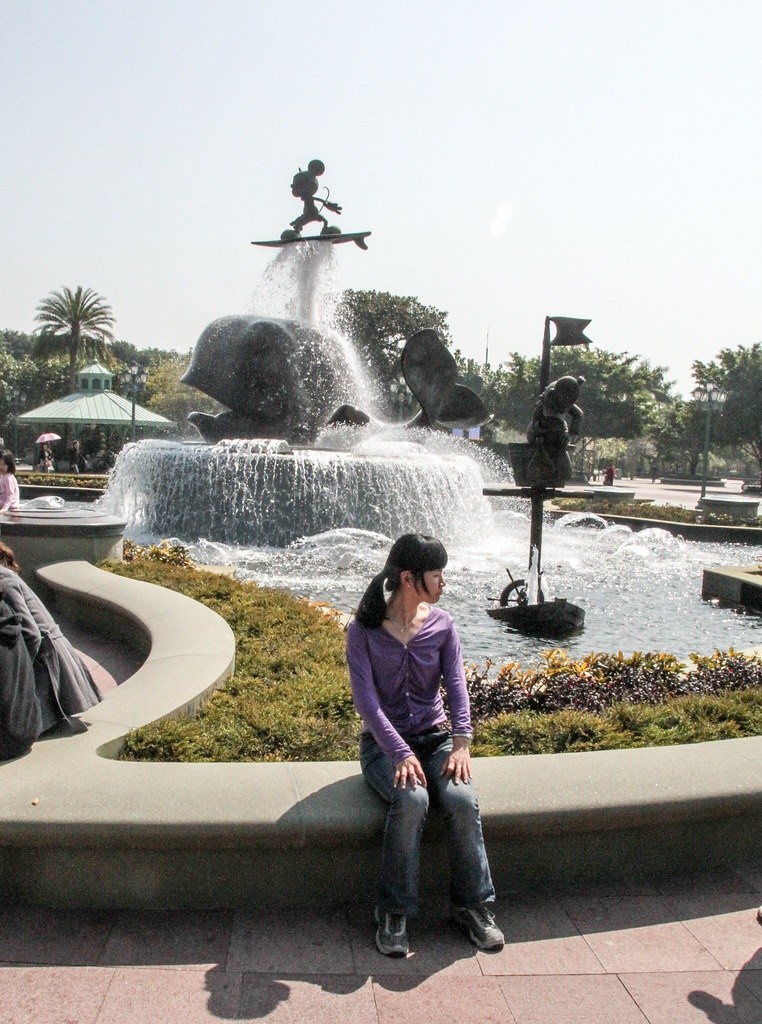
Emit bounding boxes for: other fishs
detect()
[177,315,496,444]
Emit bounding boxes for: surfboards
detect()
[250,231,373,251]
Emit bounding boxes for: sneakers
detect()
[374,904,410,957]
[449,905,505,950]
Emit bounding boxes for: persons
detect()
[345,533,504,958]
[526,375,586,480]
[39,443,52,472]
[0,543,104,758]
[0,450,20,513]
[69,439,87,474]
[603,463,616,486]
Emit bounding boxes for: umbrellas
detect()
[36,433,61,449]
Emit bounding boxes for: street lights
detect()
[691,377,727,508]
[5,386,29,464]
[119,361,147,442]
